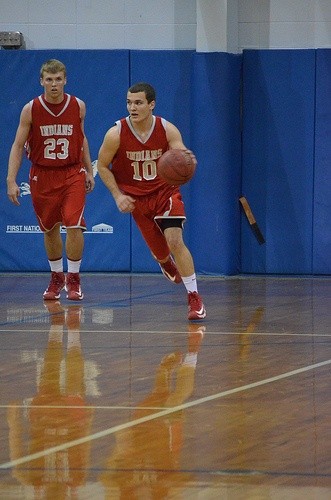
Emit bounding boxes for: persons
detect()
[6,301,95,500]
[101,321,208,500]
[98,84,206,319]
[7,60,95,301]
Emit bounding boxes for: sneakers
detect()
[157,255,182,284]
[187,290,207,321]
[42,271,66,300]
[65,272,84,301]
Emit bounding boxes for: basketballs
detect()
[157,149,195,185]
